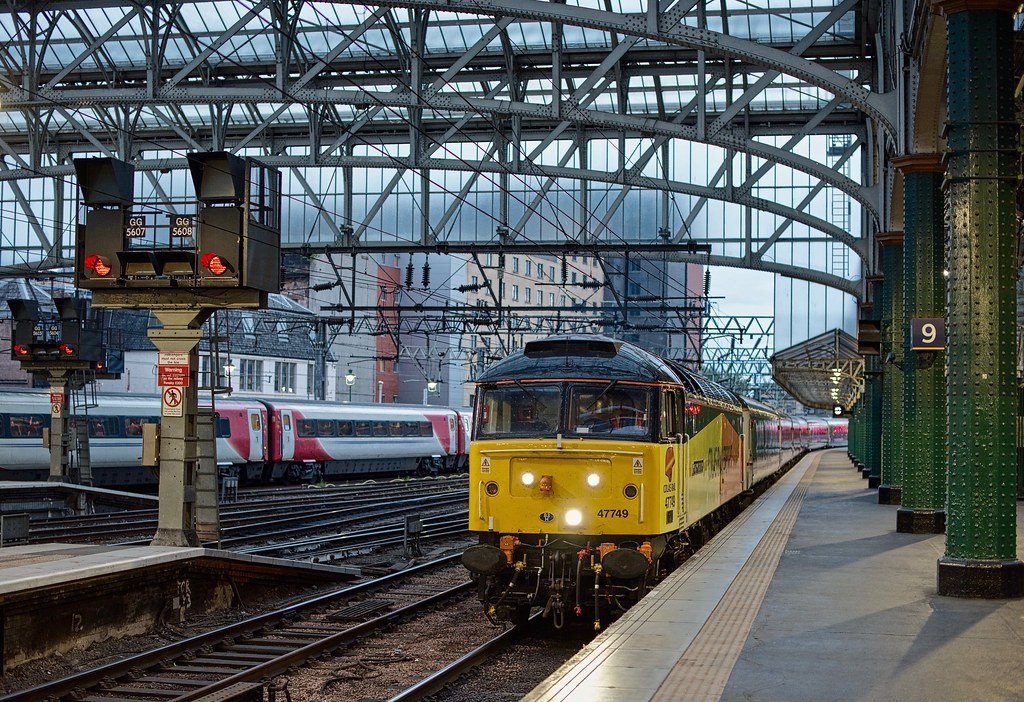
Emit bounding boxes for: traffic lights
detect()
[6,298,40,322]
[81,208,129,280]
[60,320,81,361]
[53,296,87,321]
[185,150,246,205]
[13,321,35,358]
[195,206,243,280]
[70,156,136,209]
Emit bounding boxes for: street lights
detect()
[345,367,357,401]
[222,359,237,397]
[426,379,438,405]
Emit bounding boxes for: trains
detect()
[0,384,475,487]
[457,334,849,632]
